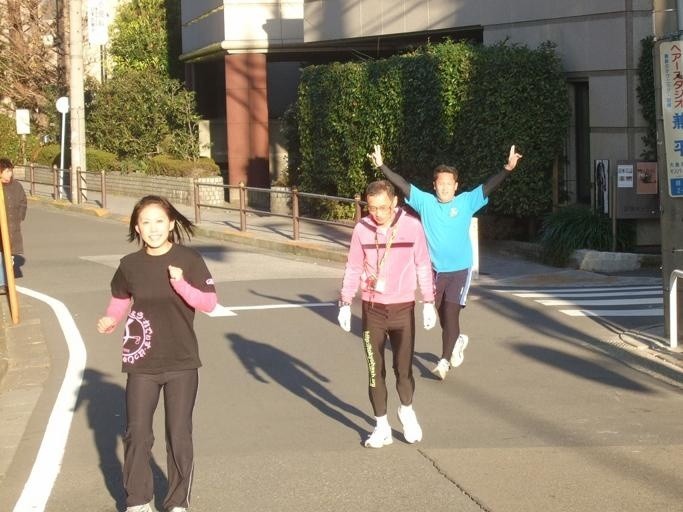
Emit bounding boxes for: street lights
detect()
[56,95,72,200]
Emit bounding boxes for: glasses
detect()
[367,200,393,212]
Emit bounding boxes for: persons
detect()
[0,156,28,295]
[98,194,218,512]
[338,178,437,450]
[372,142,523,380]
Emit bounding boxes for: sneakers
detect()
[429,358,449,380]
[124,502,152,512]
[362,426,394,449]
[448,334,469,369]
[167,506,187,512]
[394,402,423,443]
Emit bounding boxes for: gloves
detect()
[419,299,440,331]
[336,304,354,333]
